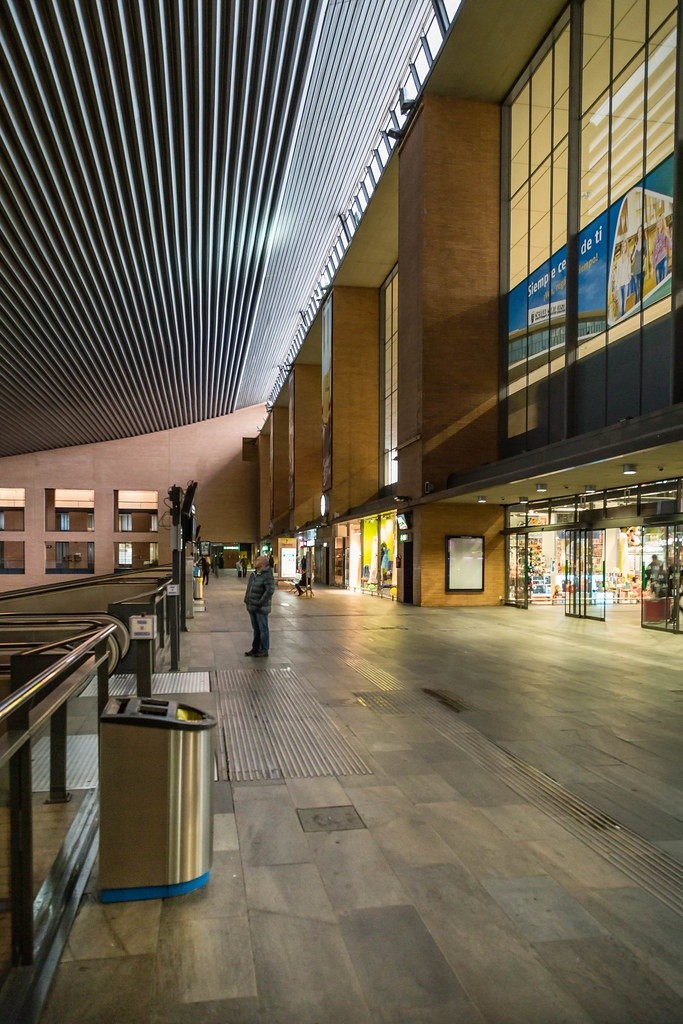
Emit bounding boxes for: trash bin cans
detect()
[99,695,217,902]
[193,576,203,600]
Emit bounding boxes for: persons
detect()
[244,556,275,658]
[196,553,224,585]
[295,554,312,597]
[236,555,249,579]
[645,555,664,589]
[629,529,641,546]
[615,213,672,318]
[264,554,274,577]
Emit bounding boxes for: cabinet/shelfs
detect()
[334,536,350,588]
[608,193,673,306]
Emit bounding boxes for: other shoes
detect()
[244,649,254,656]
[253,651,269,657]
[298,591,304,596]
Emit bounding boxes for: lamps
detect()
[394,496,412,502]
[584,484,598,494]
[623,464,637,476]
[386,128,403,140]
[476,495,489,504]
[536,484,549,493]
[397,99,418,114]
